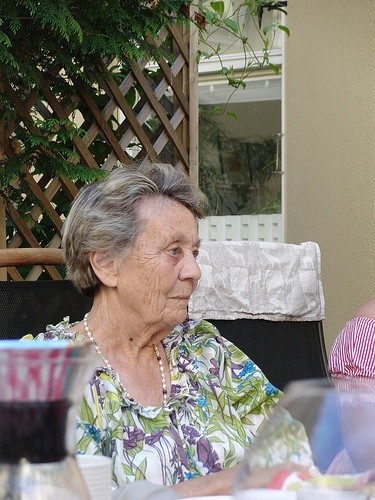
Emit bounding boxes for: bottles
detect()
[0,338,97,500]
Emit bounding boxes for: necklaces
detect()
[84,311,168,413]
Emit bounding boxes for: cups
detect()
[76,454,113,500]
[230,378,374,499]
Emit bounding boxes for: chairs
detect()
[0,248,95,341]
[185,241,348,465]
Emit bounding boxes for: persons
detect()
[328,292,375,474]
[20,163,331,499]
